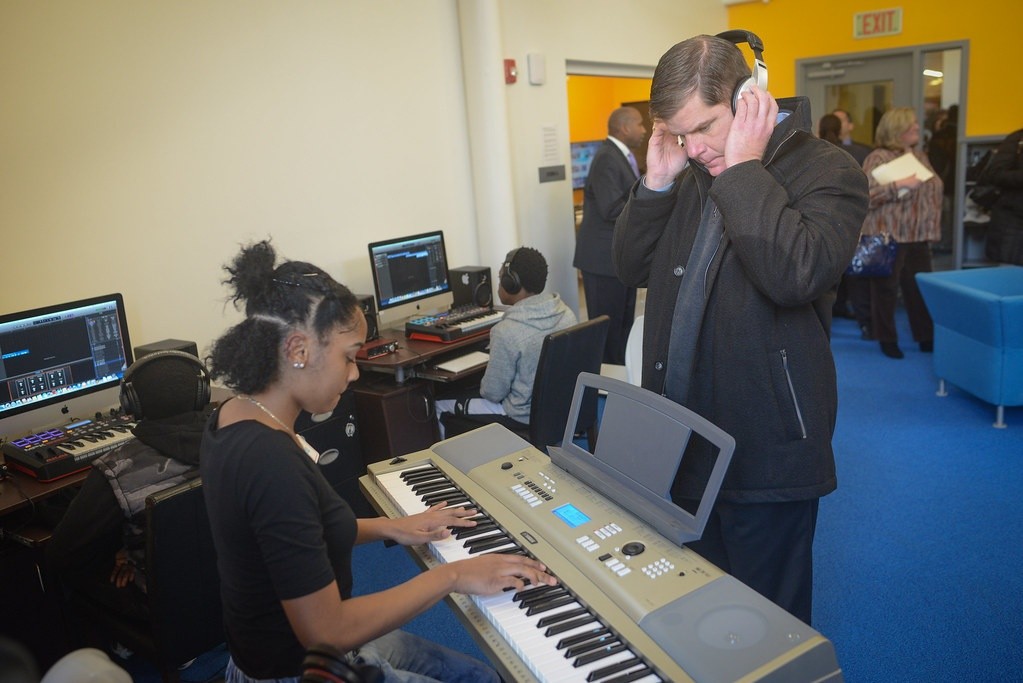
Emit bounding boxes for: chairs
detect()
[914,262,1023,428]
[68,474,211,683]
[438,314,613,454]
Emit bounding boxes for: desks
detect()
[0,386,235,583]
[349,305,513,457]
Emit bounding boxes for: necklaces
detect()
[238,394,290,430]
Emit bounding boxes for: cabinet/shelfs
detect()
[352,374,442,456]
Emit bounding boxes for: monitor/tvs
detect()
[368,230,450,331]
[0,293,136,445]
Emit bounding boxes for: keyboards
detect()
[433,350,490,373]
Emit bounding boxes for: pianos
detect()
[404,302,505,344]
[356,370,846,683]
[2,404,139,484]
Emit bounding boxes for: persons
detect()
[436,248,578,439]
[573,107,646,366]
[985,129,1023,266]
[924,105,959,252]
[862,106,944,358]
[612,34,870,623]
[44,355,212,661]
[199,246,557,683]
[815,110,871,316]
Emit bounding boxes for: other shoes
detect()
[880,341,904,358]
[861,327,875,341]
[919,341,933,352]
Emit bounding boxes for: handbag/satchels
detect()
[845,211,900,276]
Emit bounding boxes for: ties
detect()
[629,151,640,179]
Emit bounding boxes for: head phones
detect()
[119,351,213,419]
[717,28,768,117]
[501,247,522,294]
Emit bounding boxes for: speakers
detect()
[449,265,494,307]
[134,337,202,373]
[355,293,379,342]
